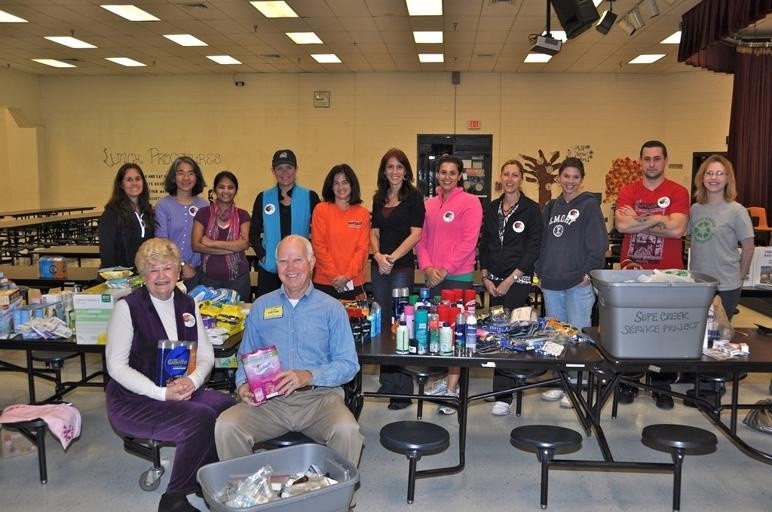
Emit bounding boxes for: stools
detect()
[698,372,748,427]
[590,367,647,419]
[30,350,79,399]
[638,422,721,512]
[0,400,75,487]
[264,431,313,448]
[494,367,548,417]
[507,424,584,512]
[393,364,450,420]
[378,419,451,506]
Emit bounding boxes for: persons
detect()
[415,155,482,415]
[100,163,154,282]
[214,234,366,468]
[192,171,253,304]
[615,142,689,410]
[370,148,426,409]
[154,156,210,293]
[535,157,609,408]
[249,150,320,298]
[480,159,542,415]
[102,237,237,512]
[311,164,371,300]
[682,157,754,408]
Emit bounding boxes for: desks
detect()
[0,206,104,296]
[581,325,772,512]
[356,320,605,512]
[1,329,244,486]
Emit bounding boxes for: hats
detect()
[271,150,296,168]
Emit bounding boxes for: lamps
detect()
[616,0,677,35]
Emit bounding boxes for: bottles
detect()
[242,345,282,402]
[157,341,197,389]
[392,285,478,357]
[32,253,69,279]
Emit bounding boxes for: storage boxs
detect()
[590,268,721,360]
[194,444,362,512]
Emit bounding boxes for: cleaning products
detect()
[702,295,722,355]
[455,305,477,355]
[440,322,453,355]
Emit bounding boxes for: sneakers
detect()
[376,379,575,416]
[618,381,723,409]
[158,493,198,512]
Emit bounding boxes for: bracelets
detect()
[513,276,517,279]
[482,276,487,281]
